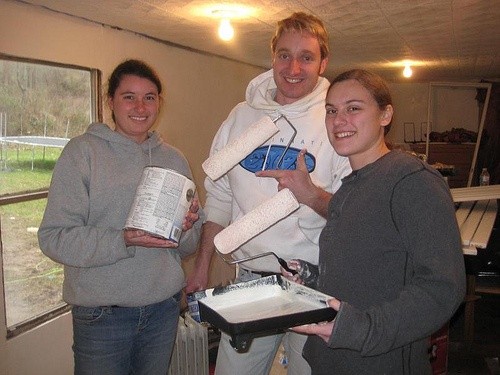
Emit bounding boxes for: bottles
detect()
[480,168,490,187]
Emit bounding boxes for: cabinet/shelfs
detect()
[411,143,477,188]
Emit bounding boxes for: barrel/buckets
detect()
[123,166,196,243]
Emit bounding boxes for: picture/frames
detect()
[403,122,415,143]
[421,122,432,142]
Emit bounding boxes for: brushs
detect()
[212,188,302,275]
[201,114,298,183]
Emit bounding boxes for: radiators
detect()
[169,313,209,375]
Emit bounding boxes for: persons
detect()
[37,59,205,375]
[183,12,354,375]
[280,69,467,375]
